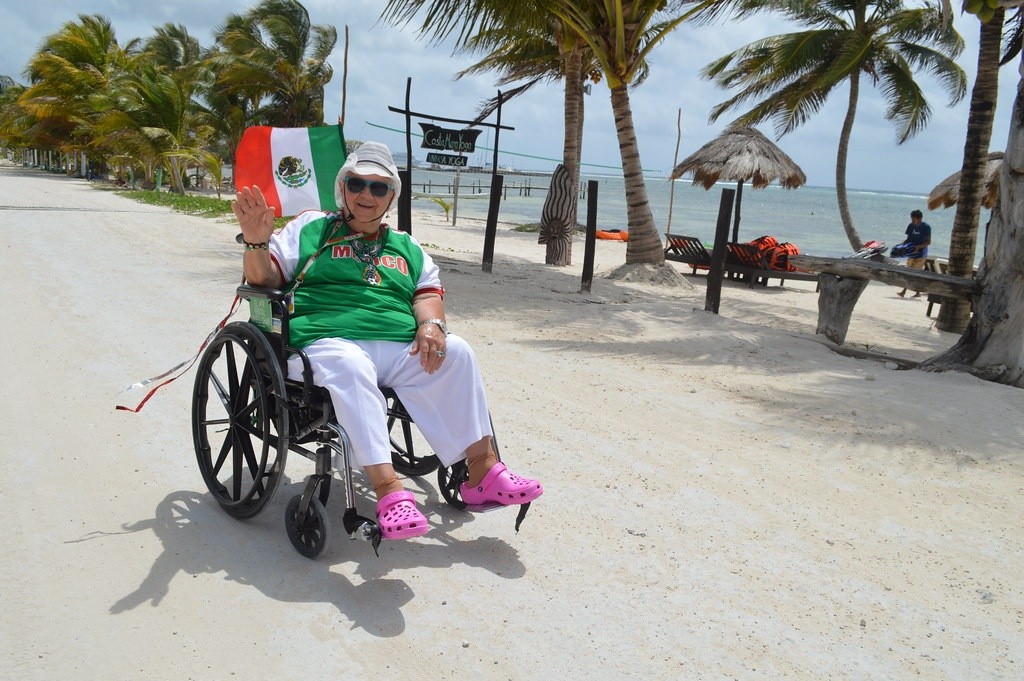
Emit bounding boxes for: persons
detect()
[897,210,931,298]
[231,141,544,540]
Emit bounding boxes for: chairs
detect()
[924,258,979,316]
[663,233,823,293]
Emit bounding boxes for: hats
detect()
[335,142,401,208]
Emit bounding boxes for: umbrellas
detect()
[668,128,806,279]
[928,151,1005,257]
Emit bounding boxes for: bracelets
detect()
[242,240,271,251]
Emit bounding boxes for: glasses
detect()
[343,175,394,197]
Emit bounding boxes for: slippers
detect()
[375,491,432,540]
[459,462,543,505]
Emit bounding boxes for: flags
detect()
[235,125,346,218]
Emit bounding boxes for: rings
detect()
[437,351,447,358]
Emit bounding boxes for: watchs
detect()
[419,319,448,337]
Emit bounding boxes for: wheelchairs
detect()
[192,284,514,559]
